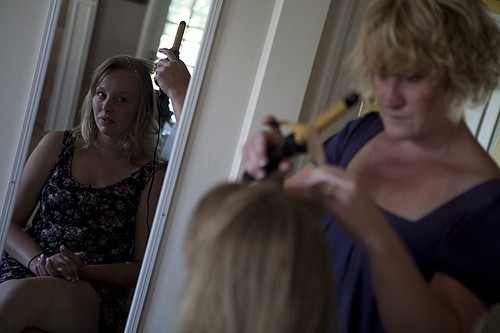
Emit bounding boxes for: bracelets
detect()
[27,252,46,272]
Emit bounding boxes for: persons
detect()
[168,177,334,333]
[239,0,500,333]
[1,47,192,333]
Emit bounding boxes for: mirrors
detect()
[0,0,224,333]
